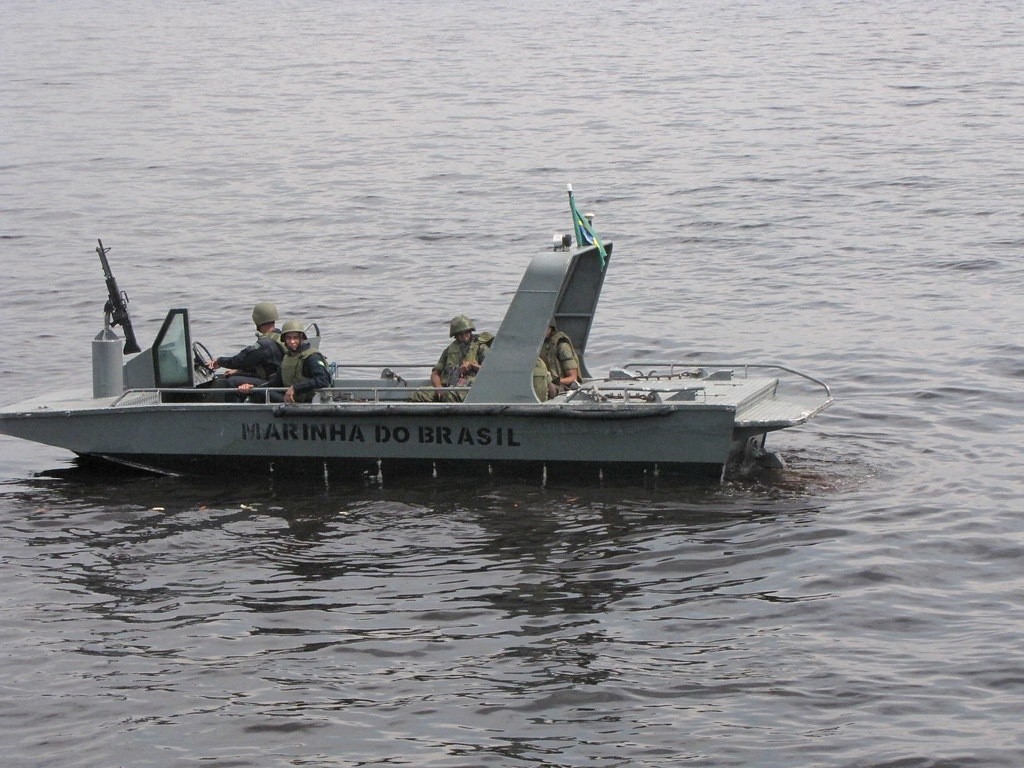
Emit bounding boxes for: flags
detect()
[570,199,607,273]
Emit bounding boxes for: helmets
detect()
[281,321,307,342]
[253,302,278,326]
[549,315,558,332]
[450,315,475,337]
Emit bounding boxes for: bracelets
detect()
[552,376,559,386]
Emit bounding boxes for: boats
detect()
[1,180,837,489]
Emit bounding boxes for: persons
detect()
[408,316,494,402]
[239,321,334,404]
[538,314,581,400]
[206,301,286,389]
